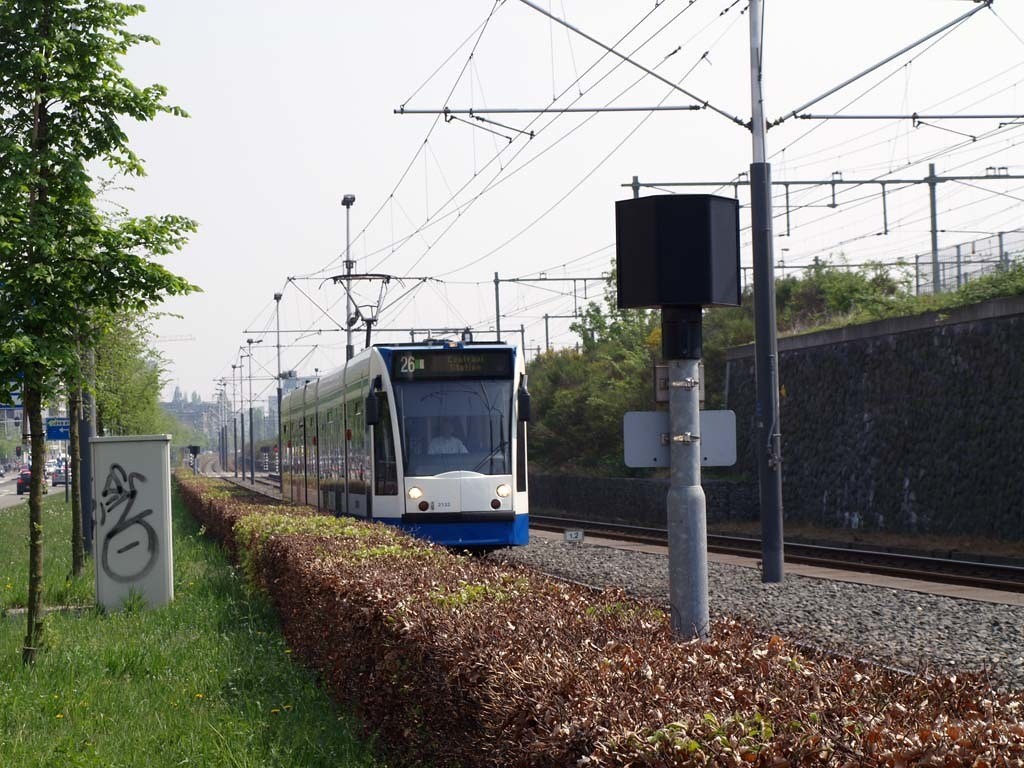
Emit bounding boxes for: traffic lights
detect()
[15,447,21,456]
[274,445,279,452]
[190,447,200,453]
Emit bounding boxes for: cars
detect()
[17,459,72,495]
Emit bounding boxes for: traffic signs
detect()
[46,418,71,440]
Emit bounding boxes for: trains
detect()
[280,273,532,556]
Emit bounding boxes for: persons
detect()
[426,422,468,455]
